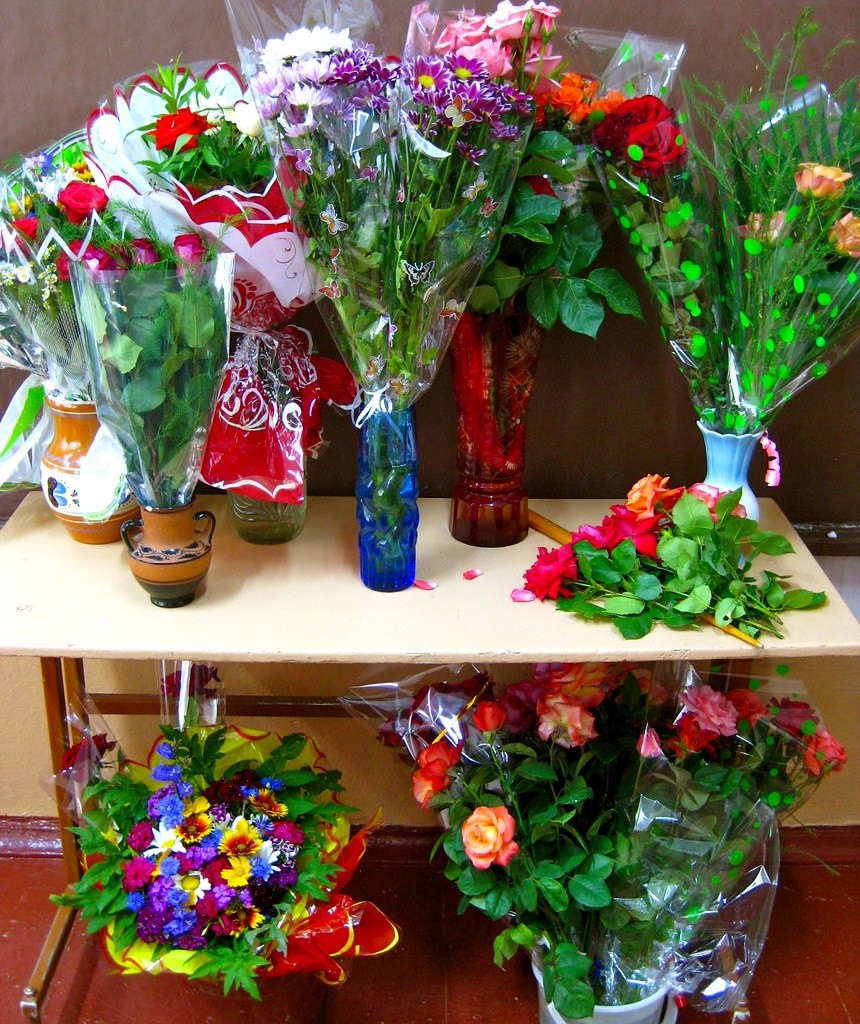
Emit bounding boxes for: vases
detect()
[696,419,769,526]
[41,389,141,545]
[528,948,680,1024]
[228,338,309,544]
[357,409,418,593]
[438,308,541,547]
[121,496,216,607]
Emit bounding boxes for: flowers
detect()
[44,662,399,994]
[0,0,860,635]
[373,663,846,1017]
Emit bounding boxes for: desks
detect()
[2,491,860,1024]
